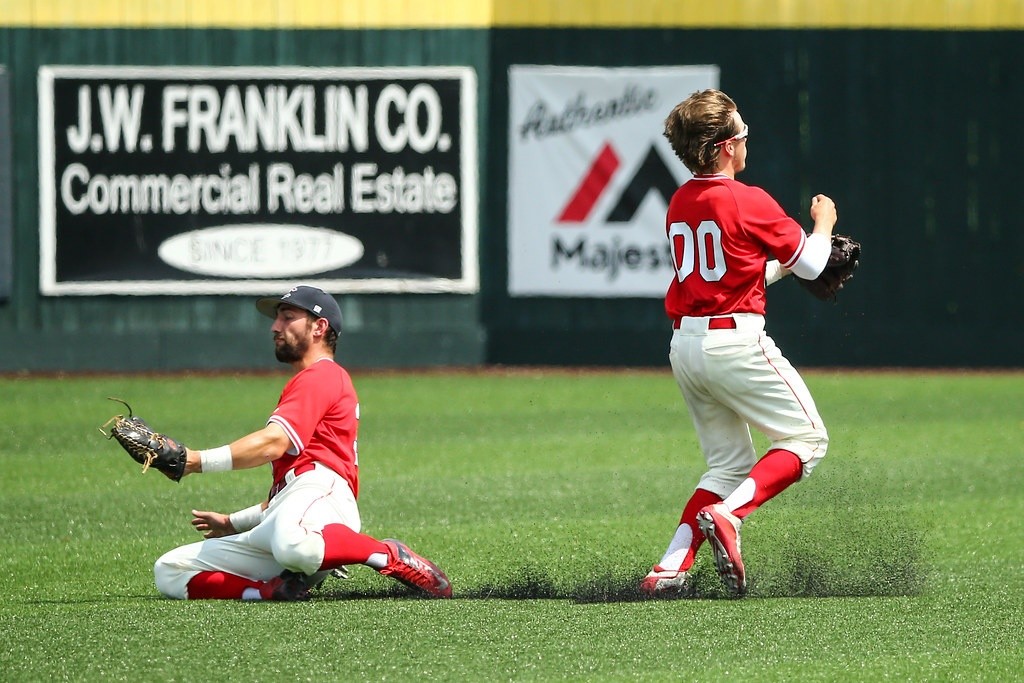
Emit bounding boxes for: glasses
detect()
[714,124,749,147]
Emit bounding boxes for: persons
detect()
[98,286,453,599]
[641,88,861,599]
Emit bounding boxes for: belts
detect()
[674,317,736,329]
[268,463,315,503]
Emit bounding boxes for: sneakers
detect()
[696,501,748,593]
[638,564,691,600]
[271,569,310,601]
[379,537,453,599]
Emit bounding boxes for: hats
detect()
[255,286,343,338]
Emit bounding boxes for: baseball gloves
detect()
[97,395,186,484]
[806,233,862,303]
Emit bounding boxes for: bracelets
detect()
[199,445,233,475]
[229,503,264,534]
